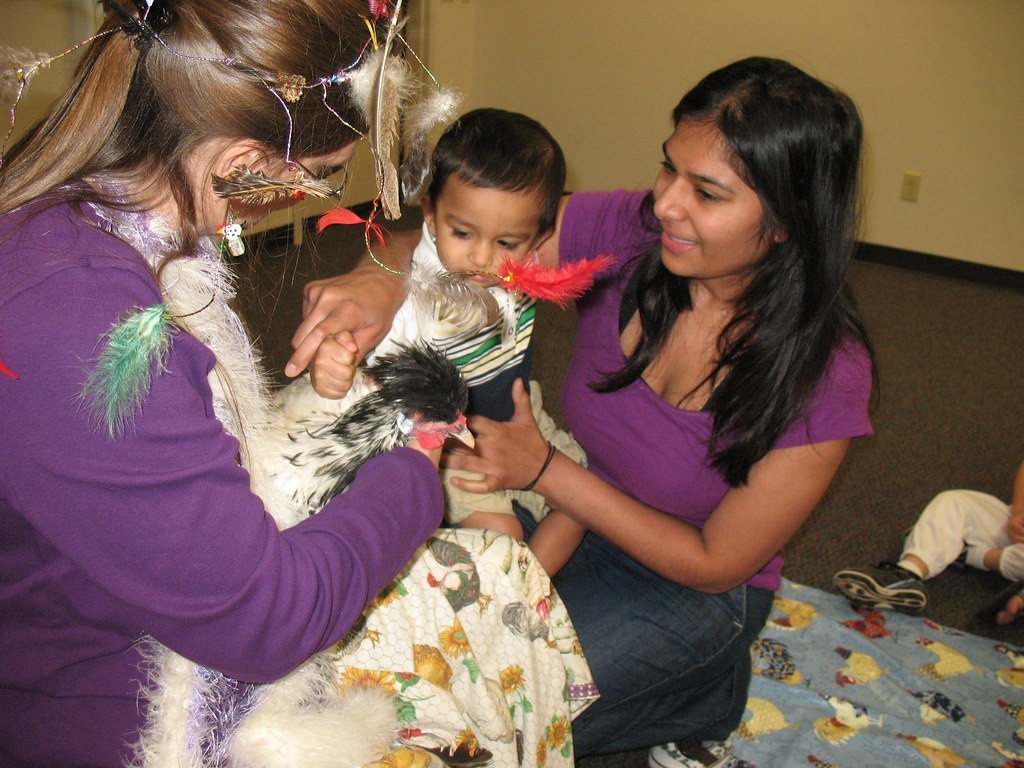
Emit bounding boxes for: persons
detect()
[298,106,591,579]
[1,1,444,766]
[833,458,1024,624]
[285,55,883,768]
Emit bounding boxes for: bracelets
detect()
[521,441,557,491]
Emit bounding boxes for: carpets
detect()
[726,576,1023,768]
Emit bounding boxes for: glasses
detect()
[257,140,347,201]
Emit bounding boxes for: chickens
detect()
[262,333,477,527]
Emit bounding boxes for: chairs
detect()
[241,101,400,249]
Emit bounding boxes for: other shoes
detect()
[901,527,970,568]
[832,562,927,609]
